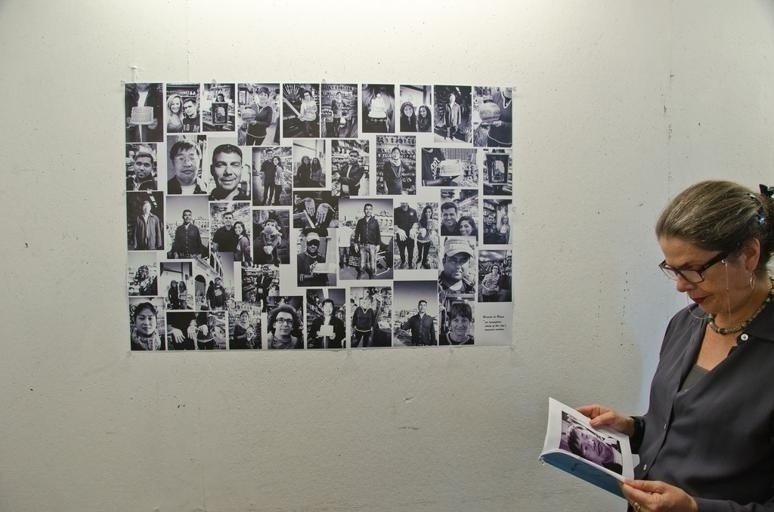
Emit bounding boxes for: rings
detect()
[636,504,643,512]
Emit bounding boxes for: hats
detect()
[307,232,321,242]
[444,236,473,257]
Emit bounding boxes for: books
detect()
[539,393,636,504]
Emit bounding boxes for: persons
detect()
[573,176,773,509]
[125,85,510,352]
[561,411,624,476]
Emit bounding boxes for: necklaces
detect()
[707,281,772,334]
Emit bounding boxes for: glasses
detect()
[659,251,730,284]
